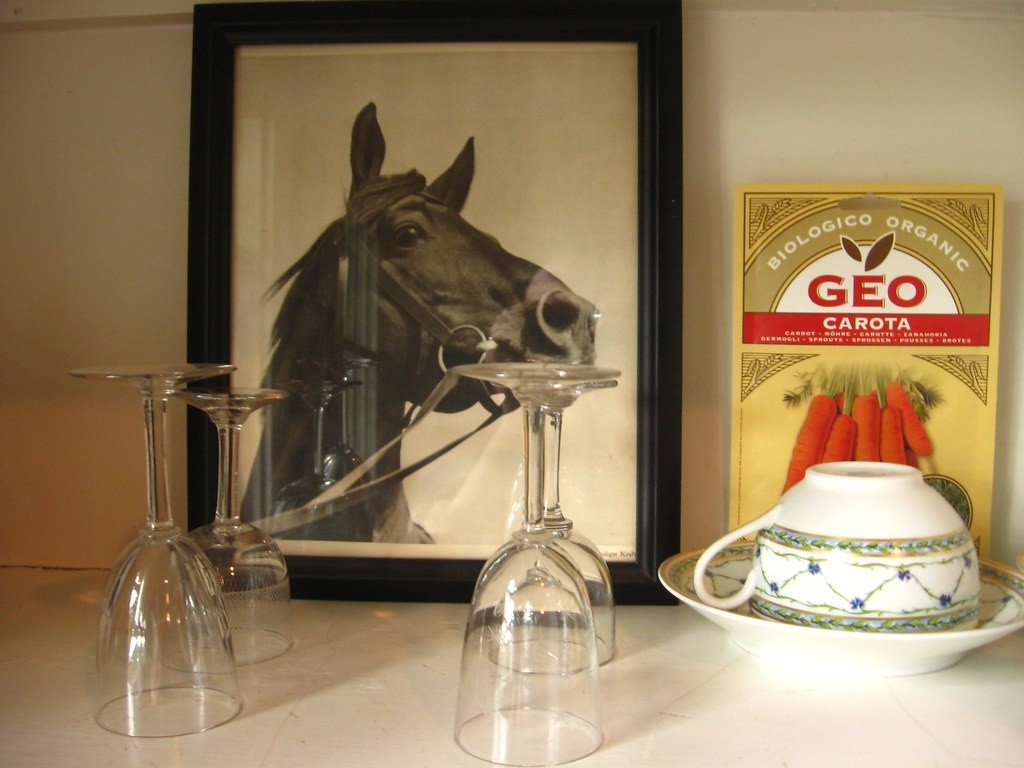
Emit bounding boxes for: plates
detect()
[658,541,1023,677]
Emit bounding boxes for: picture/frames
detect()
[186,0,682,609]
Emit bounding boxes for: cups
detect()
[692,459,980,634]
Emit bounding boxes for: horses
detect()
[239,101,600,543]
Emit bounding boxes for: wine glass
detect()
[166,388,295,666]
[70,365,244,740]
[448,362,623,767]
[264,376,374,542]
